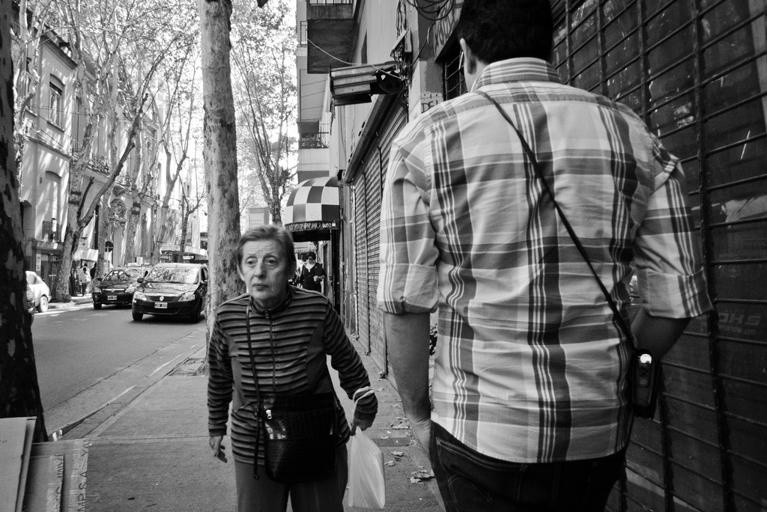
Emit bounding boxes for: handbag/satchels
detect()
[255,390,340,476]
[629,349,663,423]
[342,425,386,512]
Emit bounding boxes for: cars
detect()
[92,262,208,323]
[25,271,52,325]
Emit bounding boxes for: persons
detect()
[207,225,378,512]
[79,261,97,297]
[377,0,715,512]
[300,252,326,293]
